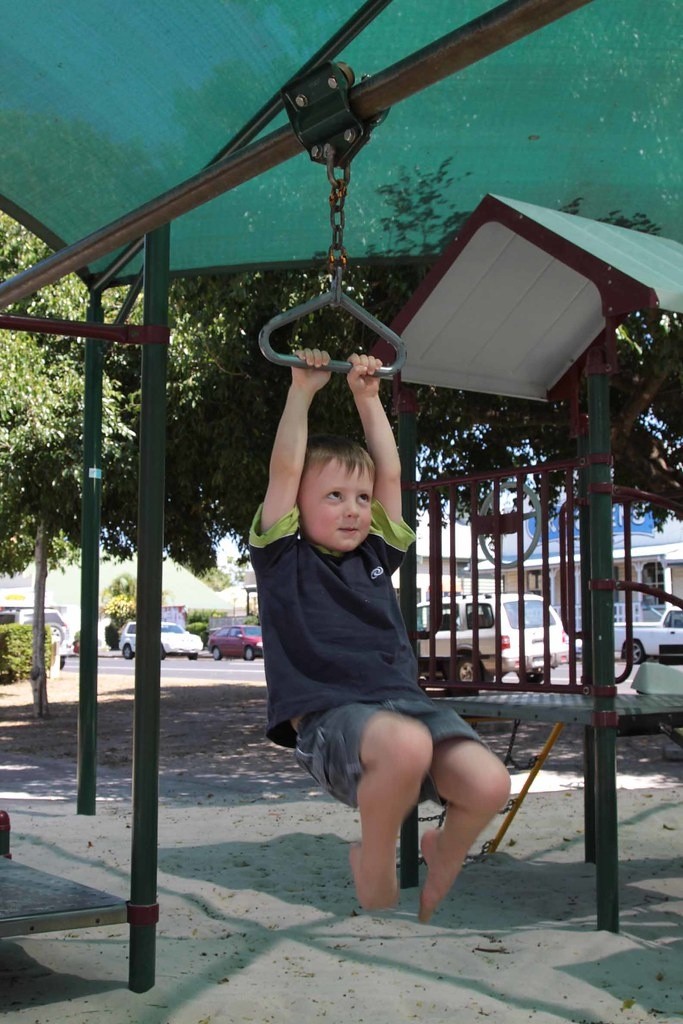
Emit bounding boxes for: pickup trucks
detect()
[612,606,683,665]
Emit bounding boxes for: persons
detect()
[248,348,511,923]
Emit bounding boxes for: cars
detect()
[208,624,264,661]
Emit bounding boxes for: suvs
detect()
[119,620,204,660]
[417,591,570,688]
[0,610,75,669]
